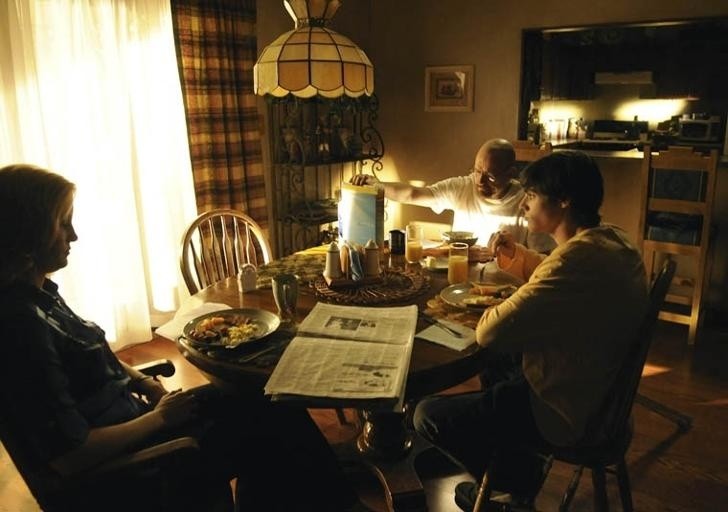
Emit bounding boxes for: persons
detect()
[412,149,647,512]
[0,166,362,512]
[348,138,552,259]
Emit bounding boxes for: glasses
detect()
[468,165,509,183]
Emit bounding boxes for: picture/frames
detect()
[424,63,475,112]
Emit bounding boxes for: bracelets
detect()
[133,375,150,392]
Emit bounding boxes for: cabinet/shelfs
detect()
[262,94,384,260]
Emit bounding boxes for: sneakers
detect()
[455,480,509,512]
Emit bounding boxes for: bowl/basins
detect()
[443,232,478,248]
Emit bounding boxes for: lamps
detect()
[253,1,374,99]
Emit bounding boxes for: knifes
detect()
[239,337,290,365]
[418,310,462,338]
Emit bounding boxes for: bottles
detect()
[326,241,343,278]
[404,224,423,265]
[271,273,299,319]
[364,239,383,276]
[447,242,469,285]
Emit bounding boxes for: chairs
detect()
[179,209,273,294]
[637,145,720,346]
[1,359,204,512]
[471,259,675,511]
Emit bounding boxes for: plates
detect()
[439,282,497,312]
[419,257,448,273]
[182,308,280,351]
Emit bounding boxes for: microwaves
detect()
[678,114,720,141]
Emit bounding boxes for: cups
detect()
[388,230,405,254]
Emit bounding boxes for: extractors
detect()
[541,22,728,99]
